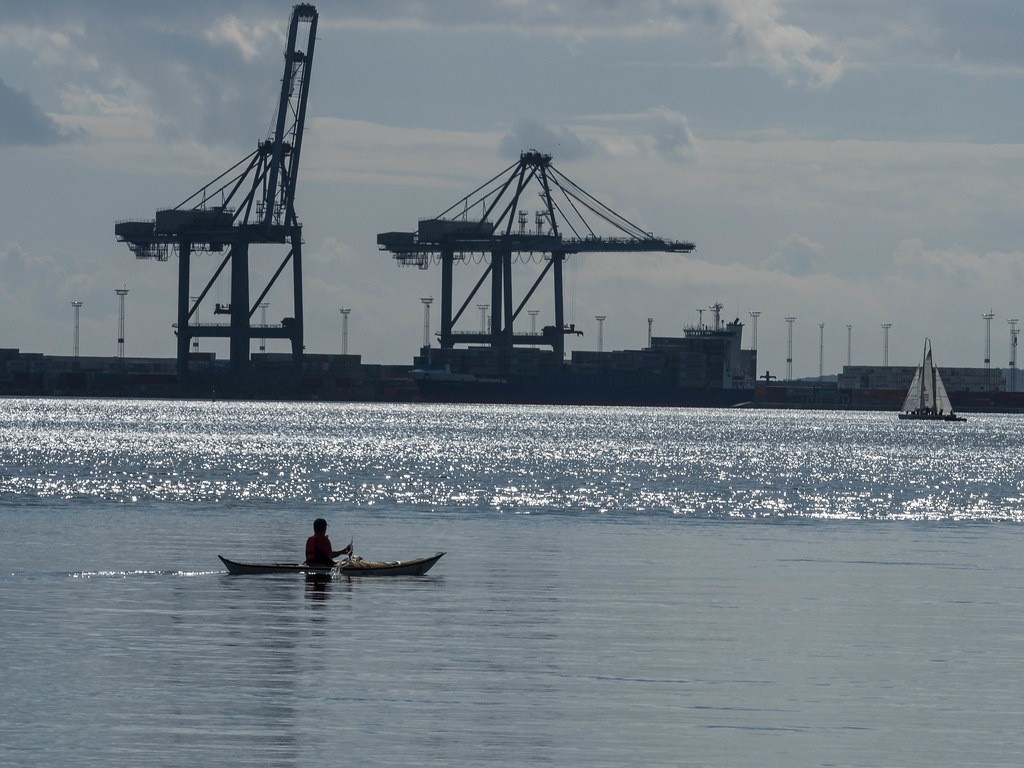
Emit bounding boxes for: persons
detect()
[306,519,353,566]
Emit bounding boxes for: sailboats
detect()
[896,337,967,422]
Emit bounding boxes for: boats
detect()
[217,551,447,575]
